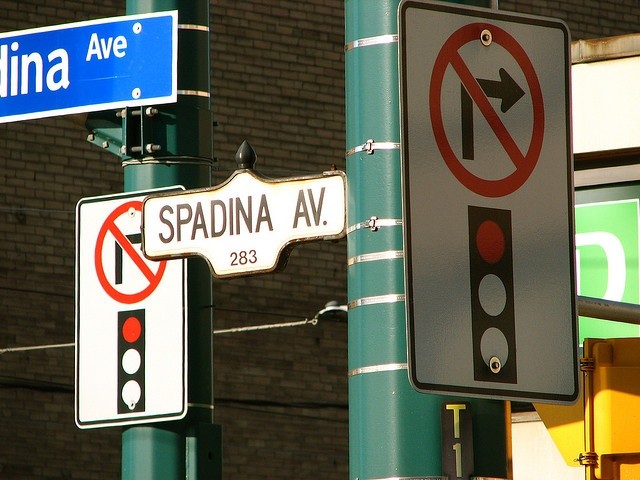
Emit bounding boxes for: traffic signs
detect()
[144,142,347,279]
[0,10,177,124]
